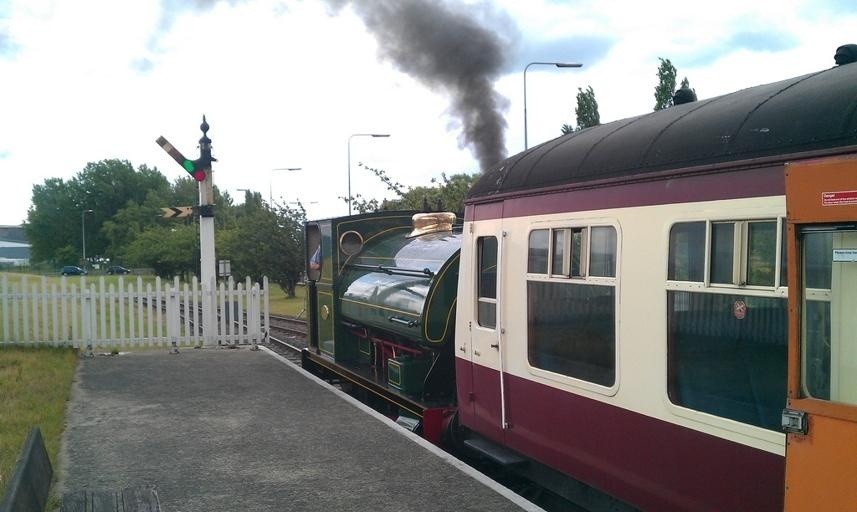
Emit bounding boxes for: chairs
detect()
[670,307,791,431]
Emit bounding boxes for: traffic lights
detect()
[182,160,205,181]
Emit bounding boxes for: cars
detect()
[106,265,131,275]
[61,265,88,276]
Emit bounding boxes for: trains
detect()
[302,45,857,511]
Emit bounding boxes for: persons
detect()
[308,244,321,270]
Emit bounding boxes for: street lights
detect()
[270,168,301,211]
[348,134,391,215]
[524,62,582,150]
[81,209,94,271]
